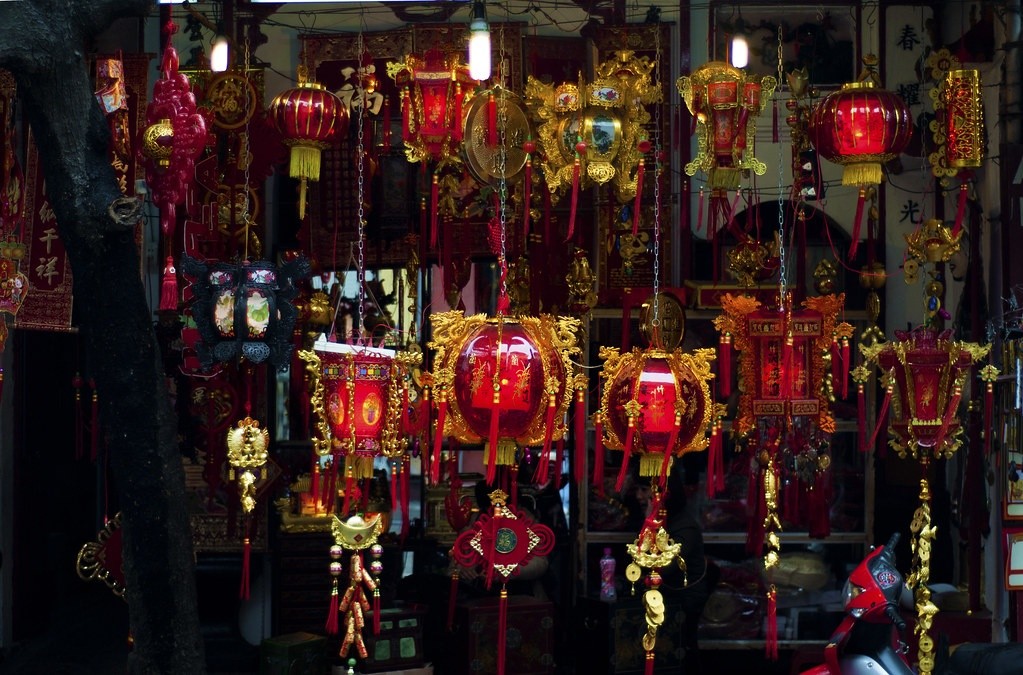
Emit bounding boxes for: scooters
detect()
[790,531,1023,675]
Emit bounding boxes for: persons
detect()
[632,464,707,675]
[948,640,1023,675]
[460,478,548,595]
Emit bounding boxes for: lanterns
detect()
[142,34,992,675]
